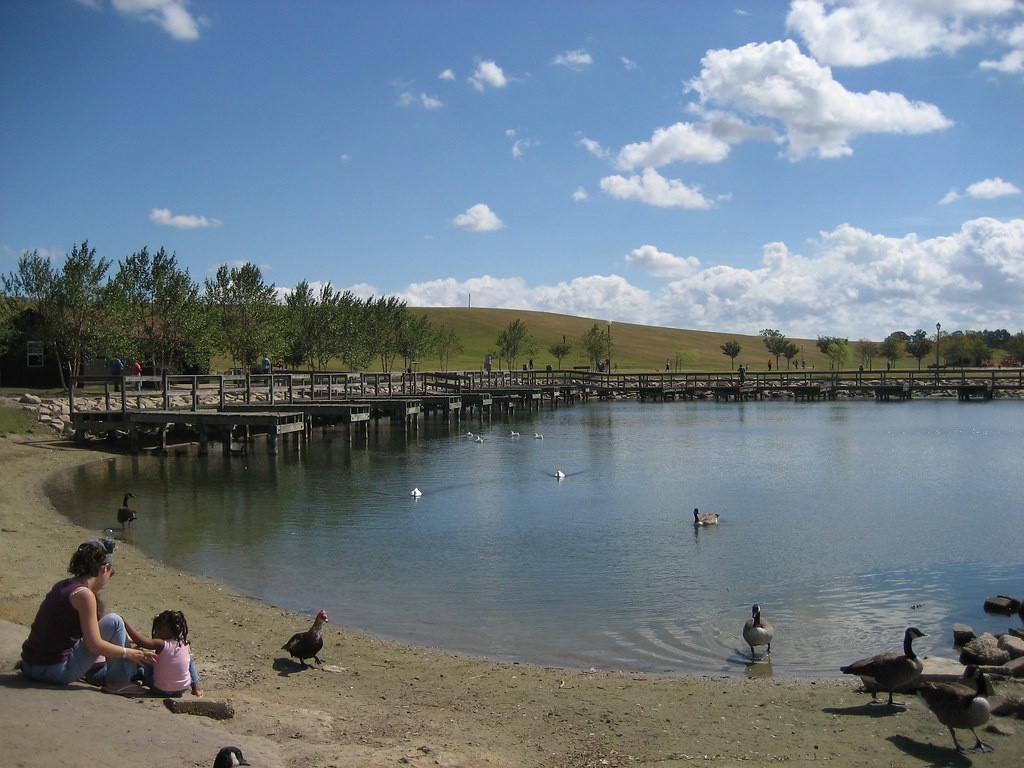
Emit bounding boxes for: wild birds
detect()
[914,663,997,756]
[475,435,484,442]
[117,492,139,530]
[693,507,720,527]
[532,432,543,441]
[409,487,422,497]
[511,430,520,438]
[836,627,929,708]
[280,610,329,671]
[467,432,473,436]
[742,603,774,654]
[554,469,566,477]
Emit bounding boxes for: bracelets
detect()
[121,646,127,660]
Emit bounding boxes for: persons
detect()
[665,358,670,372]
[18,536,204,698]
[484,354,619,373]
[262,354,271,383]
[737,364,746,386]
[112,356,124,392]
[768,359,772,371]
[793,359,806,369]
[131,360,142,390]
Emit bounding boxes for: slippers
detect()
[191,690,203,699]
[103,685,145,695]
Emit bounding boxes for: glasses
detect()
[101,565,115,577]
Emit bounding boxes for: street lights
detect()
[605,319,613,375]
[936,323,941,375]
[801,343,804,367]
